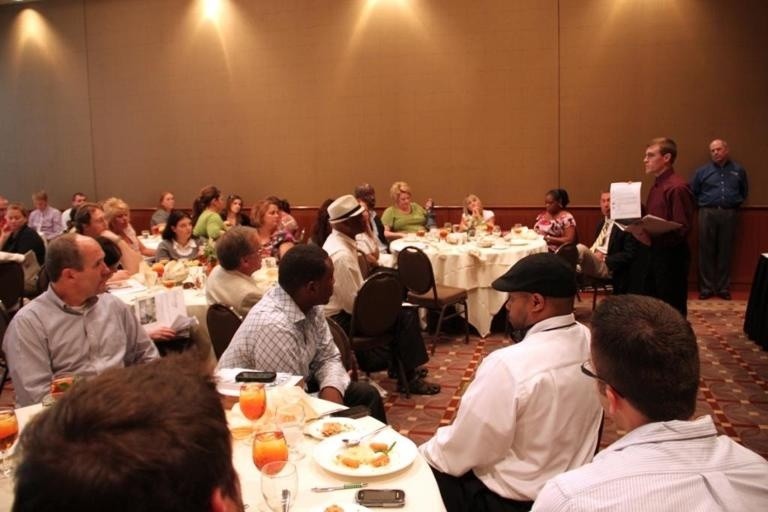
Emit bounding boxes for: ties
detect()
[596,223,608,247]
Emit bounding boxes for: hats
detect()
[327,194,365,224]
[492,252,577,298]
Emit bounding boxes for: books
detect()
[608,214,685,237]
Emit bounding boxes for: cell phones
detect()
[355,488,406,508]
[235,371,277,382]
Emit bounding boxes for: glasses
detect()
[580,361,625,399]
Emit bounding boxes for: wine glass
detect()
[260,460,299,512]
[418,222,524,248]
[240,383,266,446]
[51,371,73,399]
[252,425,289,476]
[0,403,19,453]
[193,273,206,297]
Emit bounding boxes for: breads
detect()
[341,437,389,468]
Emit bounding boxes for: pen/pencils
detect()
[311,481,368,493]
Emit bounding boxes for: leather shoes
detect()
[718,293,731,300]
[387,367,440,395]
[699,292,714,299]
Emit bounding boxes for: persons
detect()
[528,291,768,511]
[3,230,162,407]
[632,136,691,320]
[1,182,617,398]
[12,359,246,512]
[213,243,389,426]
[414,251,607,512]
[689,136,750,302]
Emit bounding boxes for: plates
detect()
[305,417,358,442]
[313,431,419,478]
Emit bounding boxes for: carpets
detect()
[315,298,765,512]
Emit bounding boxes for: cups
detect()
[161,271,176,289]
[142,230,149,241]
[144,271,157,288]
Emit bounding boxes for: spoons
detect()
[342,424,391,445]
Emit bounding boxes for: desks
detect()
[741,251,765,356]
